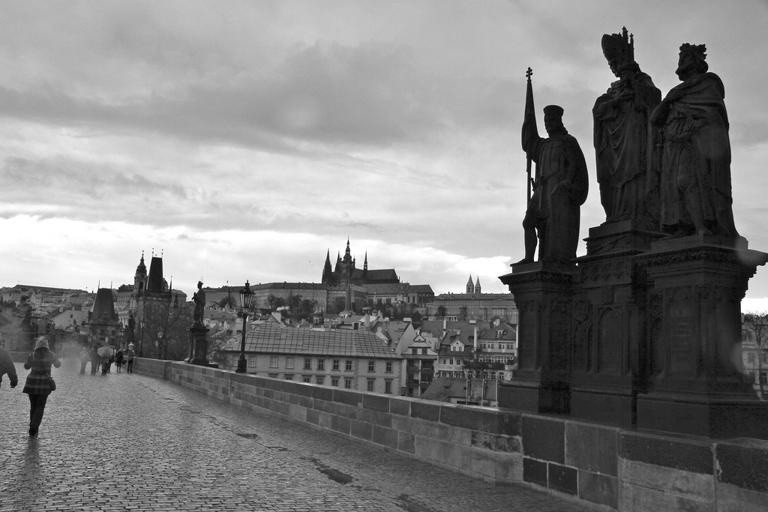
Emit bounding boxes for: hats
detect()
[33,334,50,352]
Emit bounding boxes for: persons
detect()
[519,103,590,268]
[77,342,135,377]
[191,280,206,327]
[127,314,136,337]
[0,337,18,391]
[646,42,748,242]
[591,26,663,232]
[22,334,62,439]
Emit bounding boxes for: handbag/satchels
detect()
[48,376,58,391]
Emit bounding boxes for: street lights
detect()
[236,280,256,373]
[140,317,145,357]
